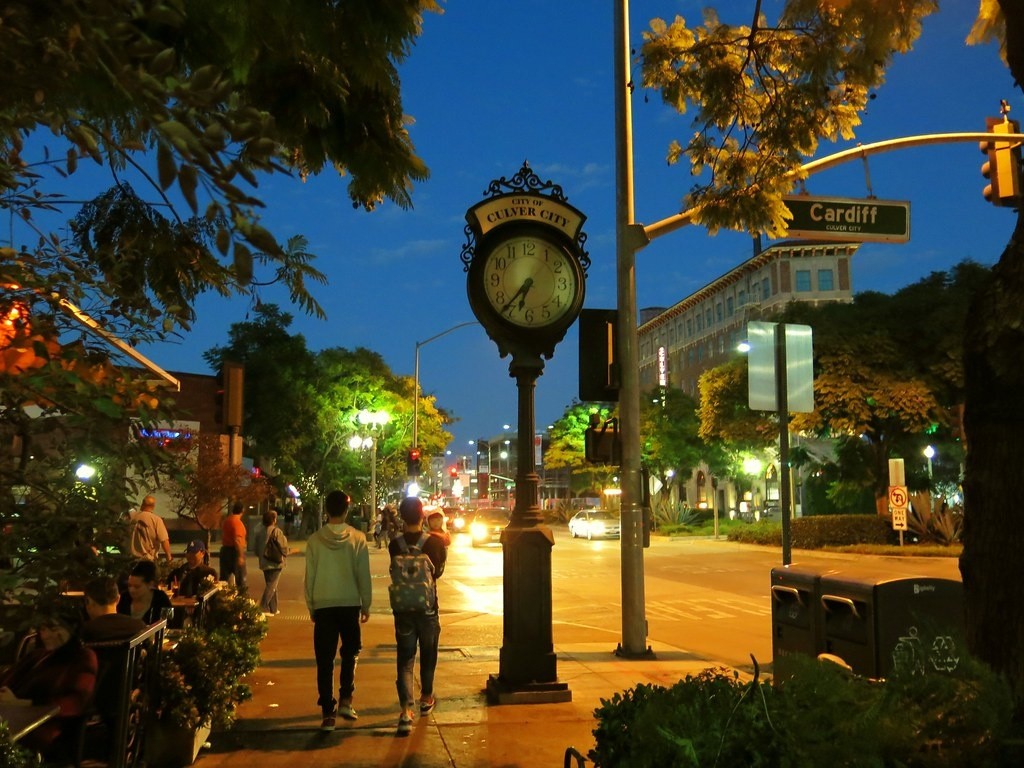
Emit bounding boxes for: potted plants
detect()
[144,593,268,764]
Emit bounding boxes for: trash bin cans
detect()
[771,559,964,682]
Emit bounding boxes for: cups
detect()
[171,582,180,600]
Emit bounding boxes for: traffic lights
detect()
[408,451,421,476]
[451,467,457,477]
[978,118,1024,208]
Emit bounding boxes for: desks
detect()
[0,701,61,743]
[169,597,200,607]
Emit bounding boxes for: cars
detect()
[471,507,511,549]
[424,505,476,533]
[568,509,620,540]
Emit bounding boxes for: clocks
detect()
[483,236,575,329]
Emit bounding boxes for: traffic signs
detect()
[892,507,908,530]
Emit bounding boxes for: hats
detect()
[184,540,206,552]
[45,597,79,619]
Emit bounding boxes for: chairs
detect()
[74,639,143,768]
[0,632,37,686]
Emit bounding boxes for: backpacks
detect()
[388,533,435,610]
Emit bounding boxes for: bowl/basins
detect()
[166,593,174,599]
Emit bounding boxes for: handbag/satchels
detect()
[263,527,283,563]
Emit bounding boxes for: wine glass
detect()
[161,608,174,636]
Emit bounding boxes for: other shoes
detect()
[320,719,336,731]
[338,704,357,720]
[419,697,436,715]
[398,712,414,731]
[275,609,280,615]
[262,612,274,617]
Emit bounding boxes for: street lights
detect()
[358,410,389,541]
[923,443,935,514]
[468,438,510,506]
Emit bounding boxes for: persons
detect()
[254,510,289,615]
[0,605,97,755]
[165,540,216,628]
[423,507,450,546]
[120,495,172,566]
[219,503,248,589]
[118,559,172,624]
[75,577,155,650]
[282,503,301,535]
[304,490,372,731]
[374,502,398,549]
[388,497,446,731]
